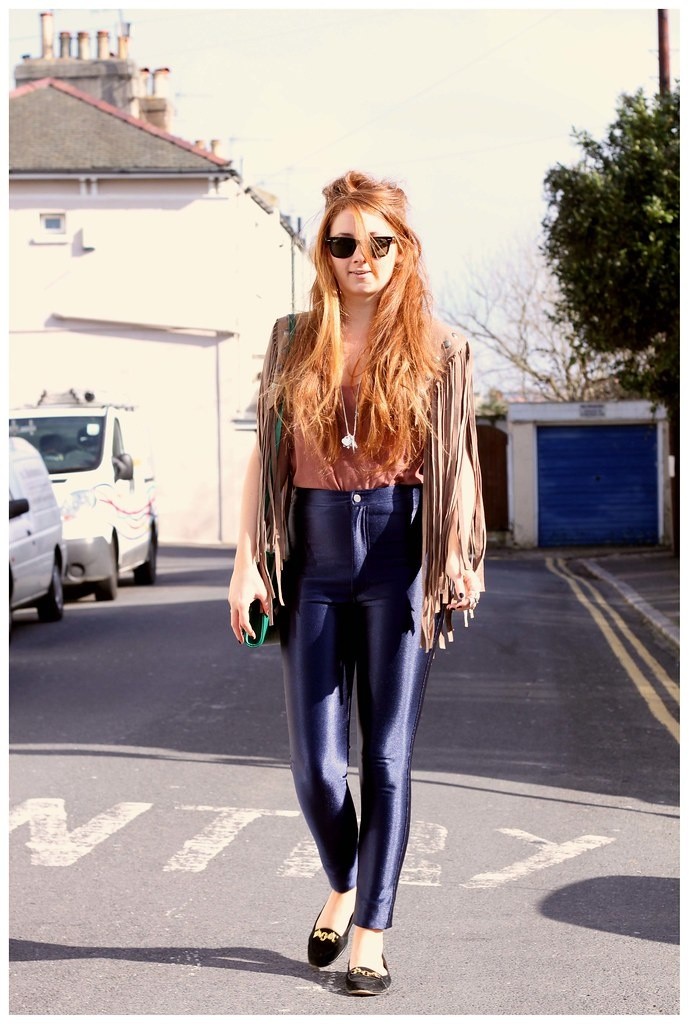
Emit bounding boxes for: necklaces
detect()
[339,378,362,452]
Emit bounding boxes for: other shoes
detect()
[346,924,392,993]
[308,883,352,968]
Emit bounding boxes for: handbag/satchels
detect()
[240,313,296,649]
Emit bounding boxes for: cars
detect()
[9,403,160,601]
[9,437,65,620]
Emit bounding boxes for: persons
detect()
[227,175,486,994]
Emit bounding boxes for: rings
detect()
[466,596,479,607]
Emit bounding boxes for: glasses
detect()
[323,234,401,260]
